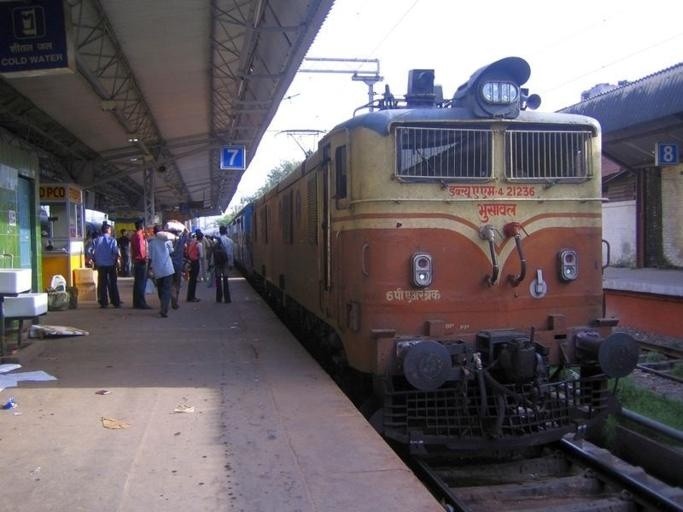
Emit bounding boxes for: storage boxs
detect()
[3,293,48,317]
[0,268,32,294]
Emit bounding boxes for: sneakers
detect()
[190,298,199,302]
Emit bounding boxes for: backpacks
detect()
[185,241,198,261]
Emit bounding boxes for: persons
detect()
[85,221,237,317]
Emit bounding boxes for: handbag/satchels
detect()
[213,238,227,264]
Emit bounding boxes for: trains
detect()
[227,60,639,454]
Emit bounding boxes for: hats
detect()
[135,220,144,227]
